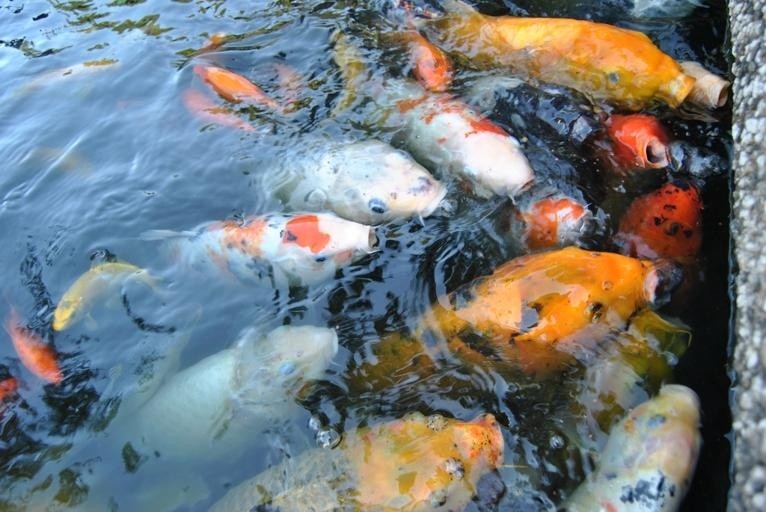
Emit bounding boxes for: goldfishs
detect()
[0,1,733,512]
